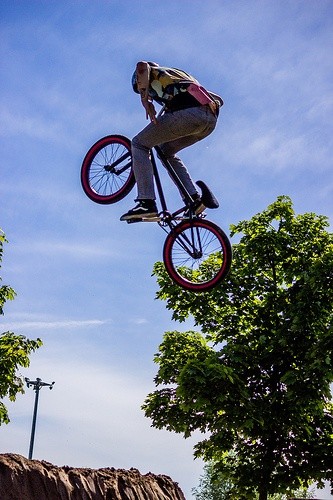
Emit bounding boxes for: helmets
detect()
[131,70,139,93]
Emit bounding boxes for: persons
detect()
[120,60,223,221]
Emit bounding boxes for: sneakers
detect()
[183,203,205,218]
[120,203,158,220]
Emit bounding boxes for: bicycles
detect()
[80,105,232,293]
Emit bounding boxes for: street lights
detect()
[24,377,55,459]
